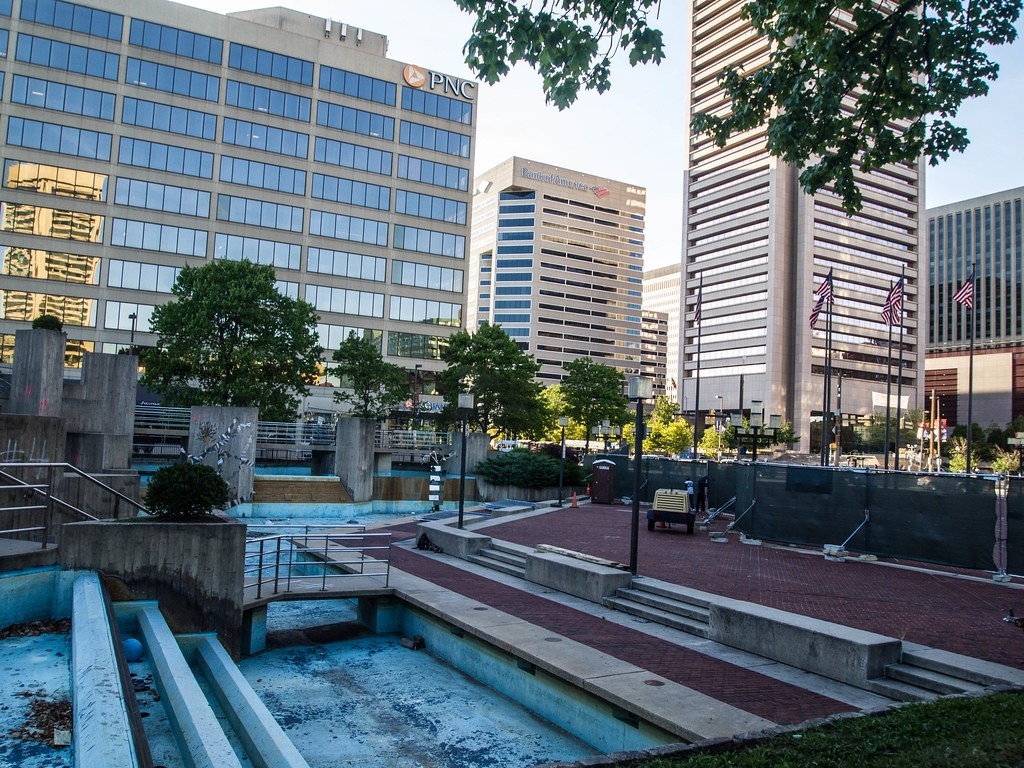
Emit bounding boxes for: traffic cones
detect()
[569,492,579,508]
[656,521,667,528]
[586,483,590,496]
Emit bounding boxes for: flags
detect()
[953,272,972,309]
[880,278,902,327]
[692,286,700,324]
[808,274,834,330]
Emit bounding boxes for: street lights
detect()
[715,396,723,461]
[730,400,782,461]
[458,393,475,529]
[591,420,621,453]
[414,364,422,392]
[627,376,653,575]
[919,411,929,471]
[128,312,136,342]
[559,416,568,504]
[930,396,940,472]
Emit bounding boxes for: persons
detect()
[695,475,710,511]
[684,476,695,512]
[428,447,438,467]
